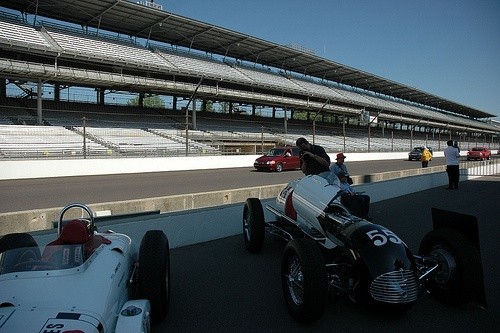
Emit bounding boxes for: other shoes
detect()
[446,187,453,190]
[455,186,459,189]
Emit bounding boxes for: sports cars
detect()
[242,172,449,315]
[0,203,172,333]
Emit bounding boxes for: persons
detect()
[329,153,353,186]
[443,140,461,189]
[295,138,330,177]
[421,146,433,168]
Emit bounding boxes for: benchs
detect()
[0,115,500,157]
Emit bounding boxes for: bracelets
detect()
[312,153,315,158]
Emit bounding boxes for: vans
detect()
[409,146,433,161]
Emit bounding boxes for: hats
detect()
[335,152,346,160]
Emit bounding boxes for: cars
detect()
[253,146,301,172]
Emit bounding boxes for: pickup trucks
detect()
[467,146,491,161]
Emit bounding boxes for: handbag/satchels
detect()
[347,178,353,185]
